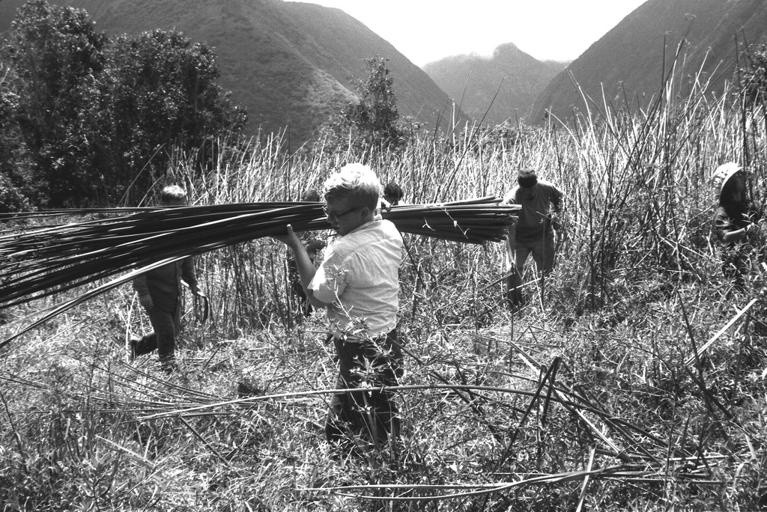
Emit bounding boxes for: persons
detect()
[711,162,766,278]
[500,168,569,312]
[128,184,202,380]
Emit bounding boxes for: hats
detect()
[713,162,743,202]
[518,168,537,187]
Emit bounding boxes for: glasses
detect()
[325,208,356,217]
